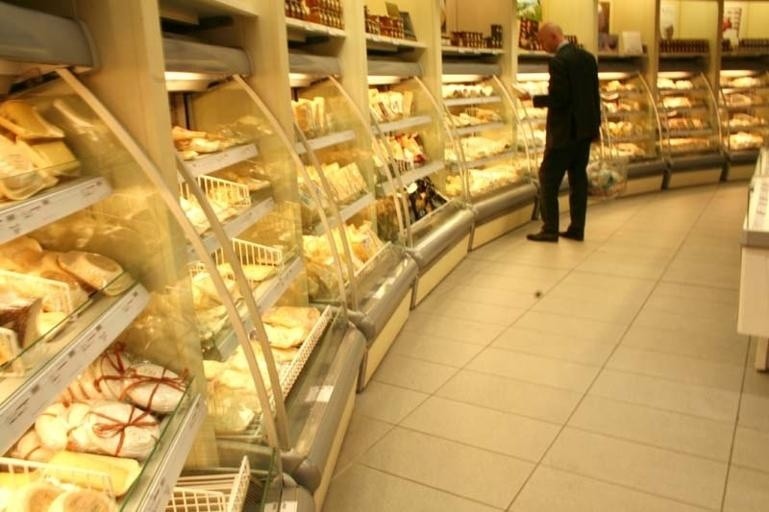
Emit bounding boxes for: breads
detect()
[291,96,381,293]
[170,117,321,433]
[0,95,188,512]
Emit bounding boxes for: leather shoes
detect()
[559,227,584,240]
[525,230,559,243]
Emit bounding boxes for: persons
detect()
[511,23,602,242]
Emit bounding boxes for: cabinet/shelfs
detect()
[1,2,362,512]
[737,146,768,374]
[284,1,537,394]
[529,3,768,210]
